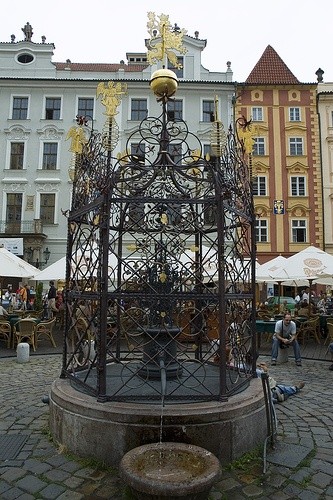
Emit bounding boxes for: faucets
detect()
[159,358,167,372]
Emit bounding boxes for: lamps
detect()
[43,247,51,263]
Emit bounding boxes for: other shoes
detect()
[296,362,301,366]
[270,360,277,365]
[297,382,304,389]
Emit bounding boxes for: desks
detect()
[10,318,41,330]
[274,314,284,317]
[6,314,18,321]
[295,316,307,321]
[251,320,278,348]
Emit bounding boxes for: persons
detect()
[0,281,36,326]
[271,314,302,366]
[295,288,331,317]
[47,280,59,312]
[56,279,84,307]
[21,22,32,41]
[230,346,306,402]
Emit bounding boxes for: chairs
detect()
[294,315,333,343]
[0,309,57,352]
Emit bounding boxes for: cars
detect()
[262,296,297,313]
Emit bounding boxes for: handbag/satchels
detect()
[280,342,288,349]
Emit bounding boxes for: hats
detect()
[269,376,276,389]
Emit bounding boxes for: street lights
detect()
[25,246,51,286]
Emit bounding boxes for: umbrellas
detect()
[0,246,41,277]
[269,245,333,319]
[229,253,317,313]
[87,243,260,305]
[29,238,139,291]
